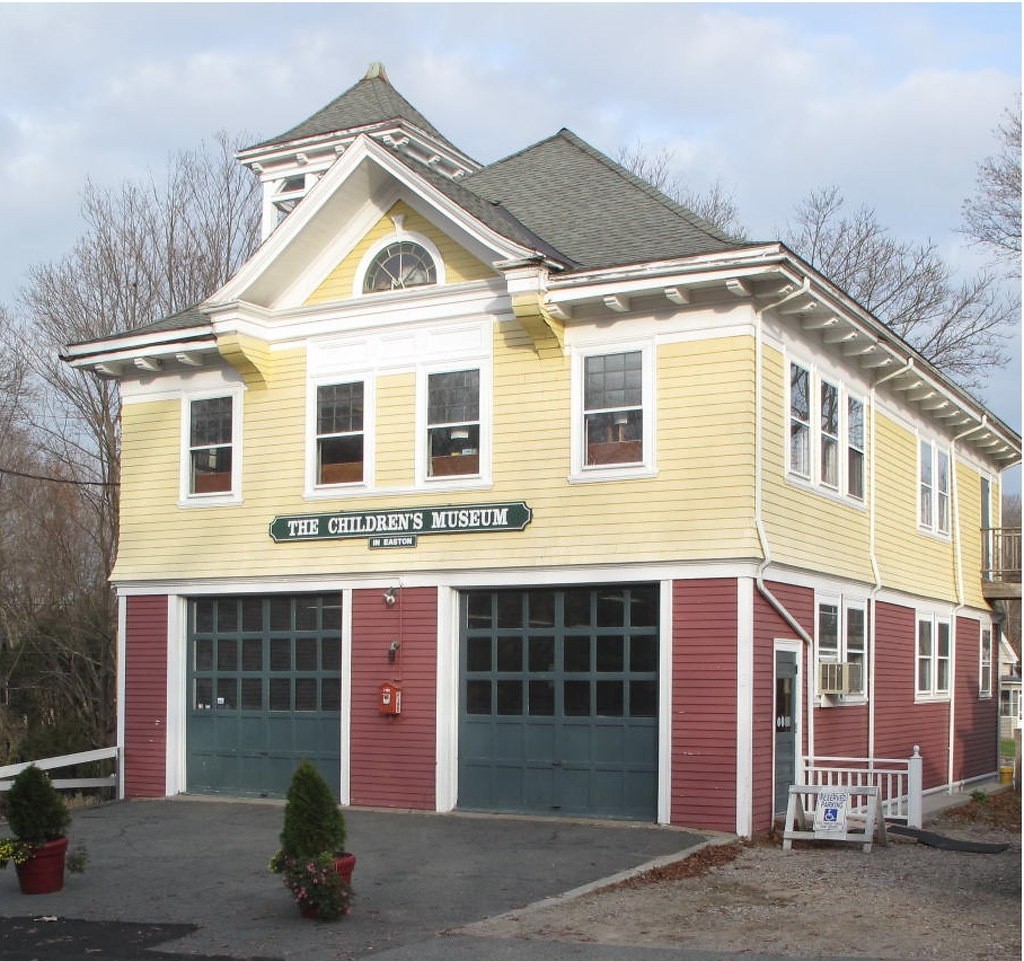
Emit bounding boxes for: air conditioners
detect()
[819,661,864,695]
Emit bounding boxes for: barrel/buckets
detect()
[1000,766,1013,784]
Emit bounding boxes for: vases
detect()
[14,836,69,894]
[301,853,355,918]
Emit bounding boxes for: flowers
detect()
[267,758,355,921]
[0,763,71,870]
